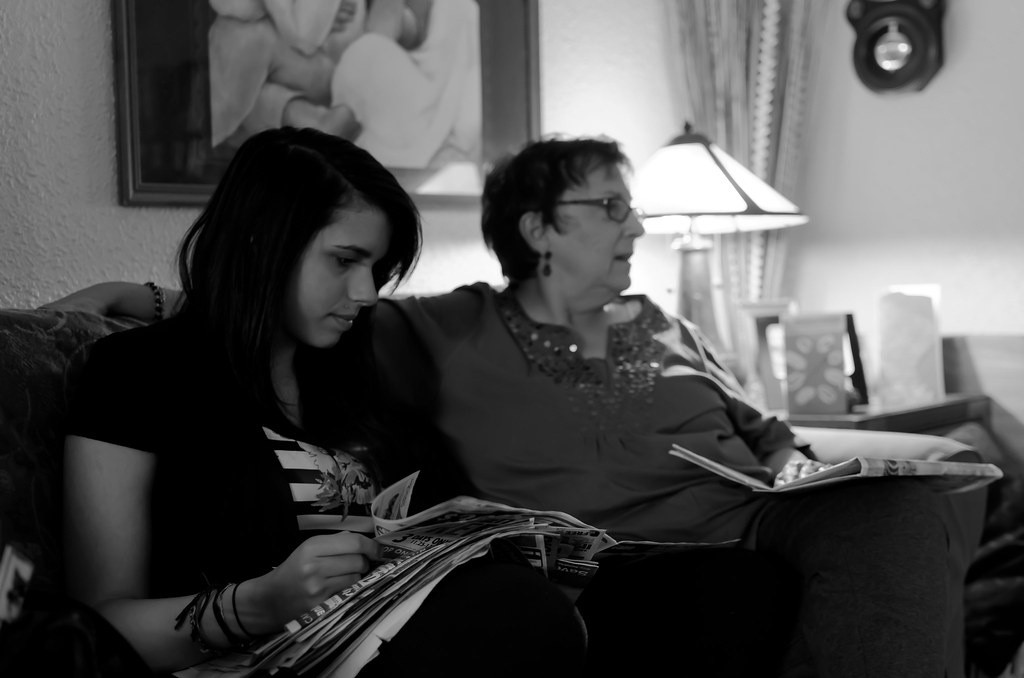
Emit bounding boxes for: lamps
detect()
[617,122,807,388]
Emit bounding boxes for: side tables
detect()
[764,390,989,490]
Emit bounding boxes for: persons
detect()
[36,139,965,678]
[210,0,434,142]
[62,125,588,678]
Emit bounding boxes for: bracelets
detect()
[175,577,256,657]
[144,282,164,320]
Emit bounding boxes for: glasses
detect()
[557,195,631,225]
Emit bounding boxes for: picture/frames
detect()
[112,0,541,211]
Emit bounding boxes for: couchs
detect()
[0,299,988,678]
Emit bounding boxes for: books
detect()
[172,471,740,678]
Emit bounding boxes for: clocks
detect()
[845,0,945,94]
